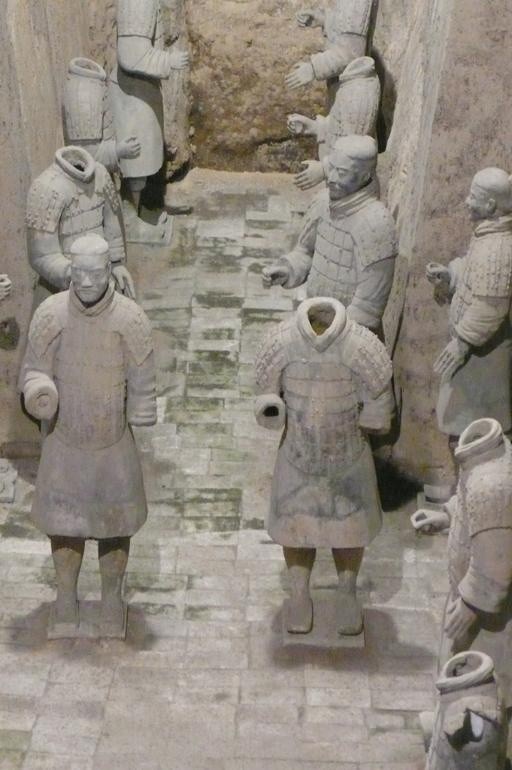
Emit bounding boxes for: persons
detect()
[285,1,373,91]
[425,167,512,495]
[286,55,383,193]
[16,233,161,621]
[61,55,142,193]
[250,294,398,635]
[409,416,512,712]
[416,650,503,770]
[261,136,400,334]
[114,1,191,228]
[16,145,139,373]
